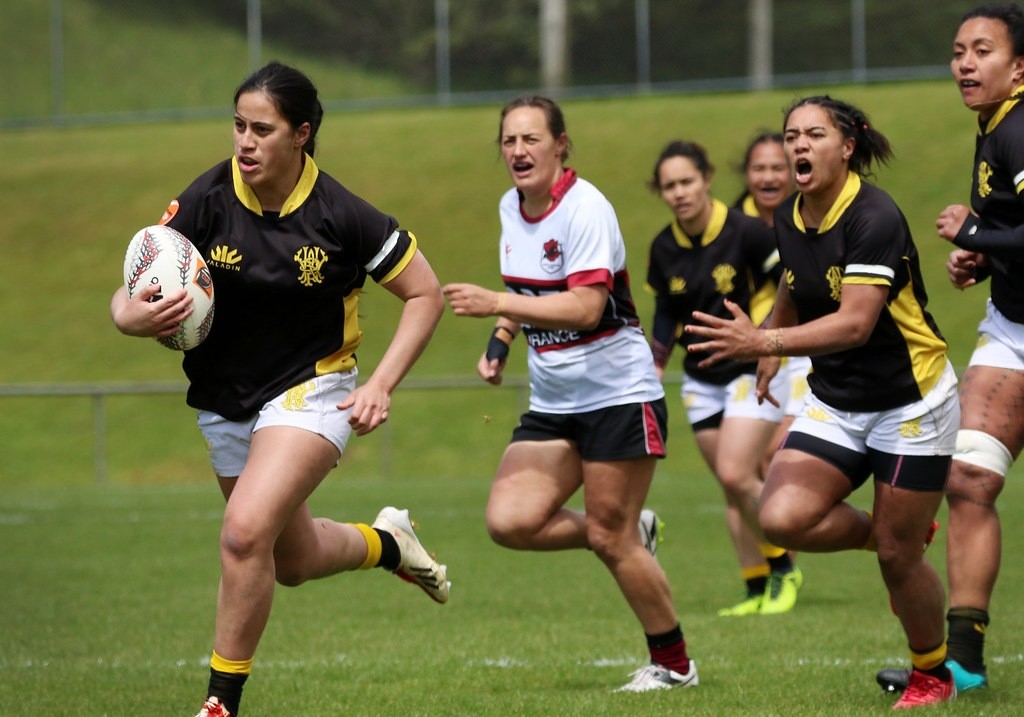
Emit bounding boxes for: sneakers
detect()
[371,505,452,603]
[636,508,666,556]
[618,659,700,693]
[759,567,804,613]
[879,656,989,695]
[891,668,957,713]
[194,695,234,717]
[722,595,765,615]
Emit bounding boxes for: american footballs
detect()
[124,224,215,352]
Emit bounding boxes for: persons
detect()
[112,61,451,717]
[441,94,699,694]
[876,6,1024,692]
[639,94,961,711]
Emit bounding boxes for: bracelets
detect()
[765,328,784,358]
